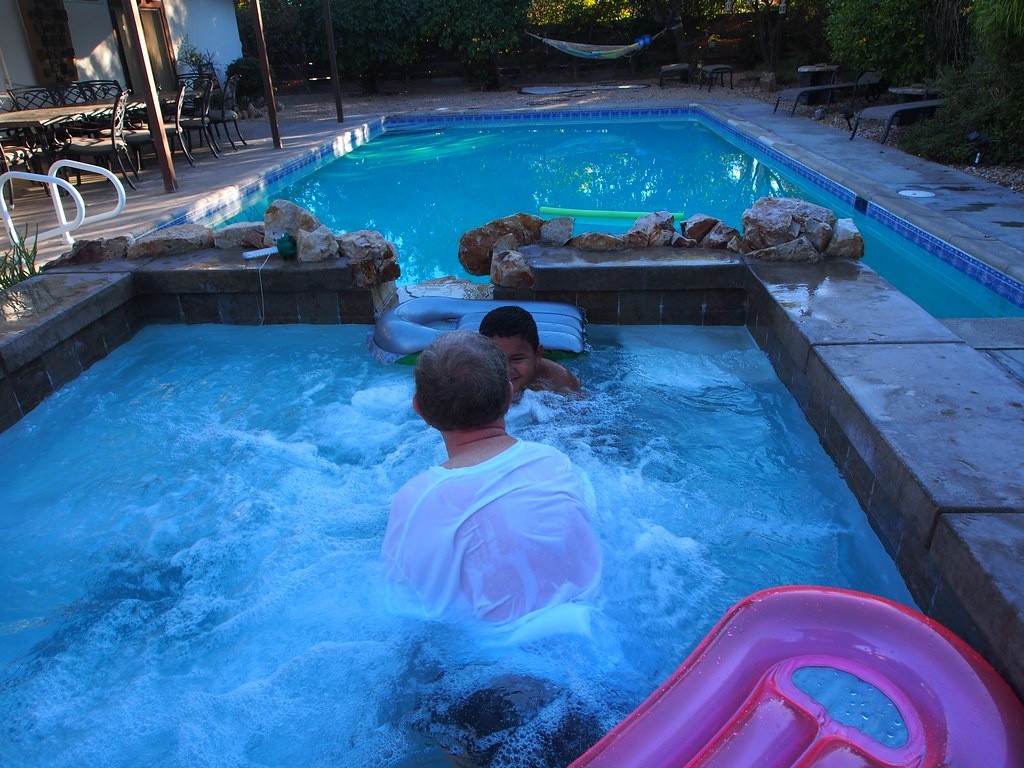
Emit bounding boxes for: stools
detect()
[658,63,692,89]
[699,64,734,92]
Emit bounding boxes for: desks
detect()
[888,86,936,126]
[0,89,222,197]
[797,66,837,105]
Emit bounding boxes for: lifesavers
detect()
[539,207,684,220]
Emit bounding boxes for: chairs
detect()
[849,82,943,144]
[0,62,248,211]
[773,65,895,116]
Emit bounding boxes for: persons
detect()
[479,306,581,401]
[382,330,603,644]
